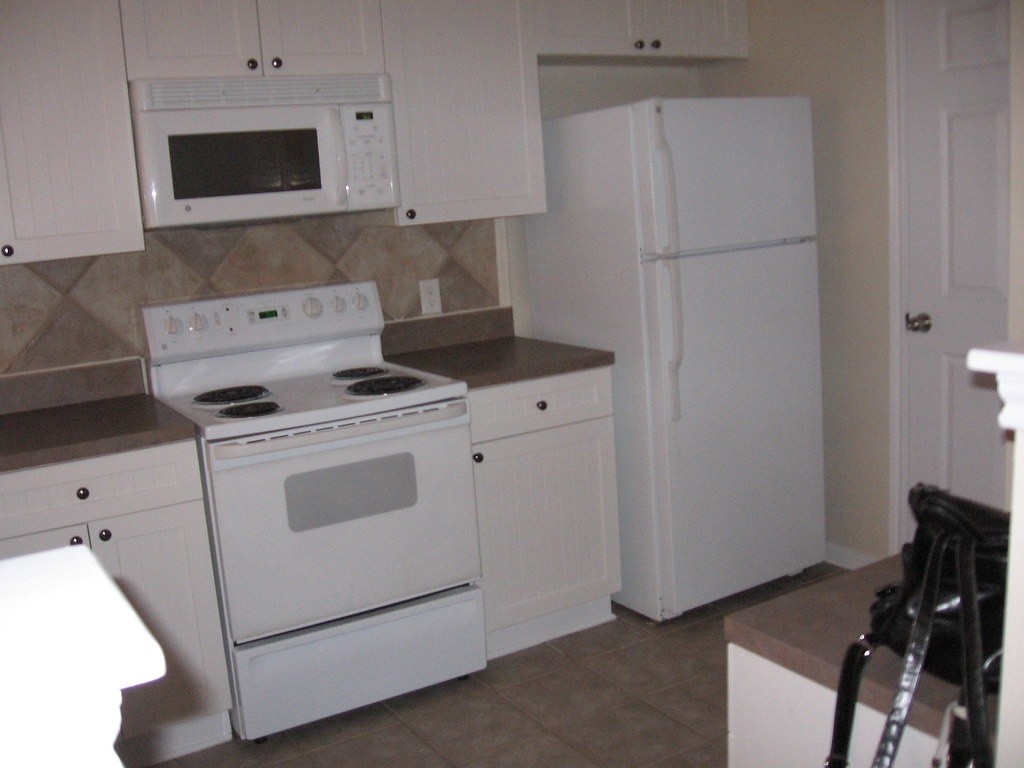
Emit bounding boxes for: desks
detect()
[722,554,960,768]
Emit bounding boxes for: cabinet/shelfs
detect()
[468,366,622,660]
[119,0,386,83]
[535,0,750,66]
[0,0,146,264]
[334,0,549,229]
[0,438,233,768]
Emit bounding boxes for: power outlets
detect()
[418,278,442,315]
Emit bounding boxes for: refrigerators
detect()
[526,96,823,621]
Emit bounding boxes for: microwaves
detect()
[130,75,400,230]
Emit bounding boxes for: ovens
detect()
[205,398,480,642]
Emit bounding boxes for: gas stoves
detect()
[144,283,467,441]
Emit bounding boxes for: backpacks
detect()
[828,483,1012,768]
